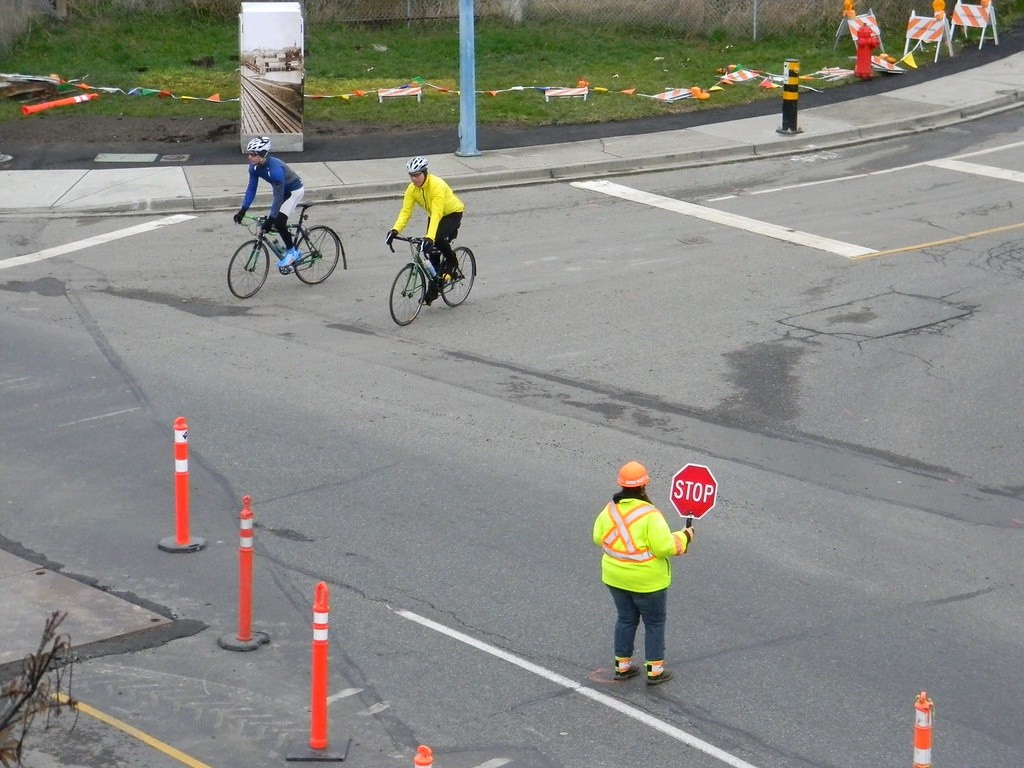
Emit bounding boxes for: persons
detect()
[593,461,695,685]
[385,157,465,305]
[233,137,304,267]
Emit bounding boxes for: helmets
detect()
[246,137,272,157]
[406,156,429,175]
[617,461,650,488]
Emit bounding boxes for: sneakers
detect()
[277,245,301,268]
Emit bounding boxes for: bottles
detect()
[424,259,436,277]
[272,237,286,254]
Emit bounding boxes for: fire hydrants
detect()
[853,20,883,78]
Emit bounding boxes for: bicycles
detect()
[224,202,343,300]
[387,234,478,327]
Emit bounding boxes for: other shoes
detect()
[418,291,438,305]
[647,670,672,684]
[444,251,458,275]
[614,664,641,679]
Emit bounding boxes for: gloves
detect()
[261,216,276,233]
[419,238,434,253]
[682,525,695,542]
[386,229,398,245]
[233,207,247,224]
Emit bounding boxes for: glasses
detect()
[247,153,258,156]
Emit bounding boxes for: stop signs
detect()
[669,463,718,520]
